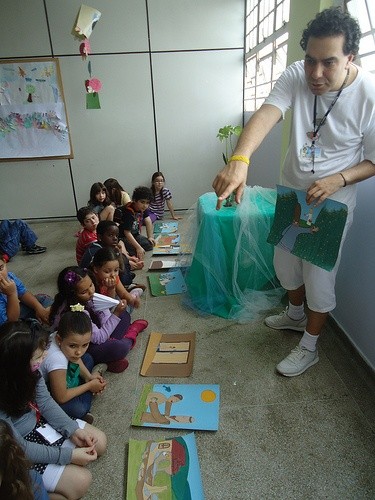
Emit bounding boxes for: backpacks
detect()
[113,202,134,223]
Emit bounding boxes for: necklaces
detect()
[311,68,350,174]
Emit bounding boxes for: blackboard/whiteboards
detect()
[0,58,74,160]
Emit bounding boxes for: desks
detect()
[181,186,279,321]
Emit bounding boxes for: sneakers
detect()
[264,306,307,332]
[276,345,319,377]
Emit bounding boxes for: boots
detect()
[123,319,148,350]
[101,338,129,373]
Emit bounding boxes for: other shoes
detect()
[127,284,146,293]
[21,243,47,255]
[130,288,143,298]
[90,363,107,377]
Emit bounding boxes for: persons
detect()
[38,303,108,424]
[80,220,136,285]
[0,418,51,500]
[76,178,156,271]
[0,218,47,263]
[0,320,106,500]
[142,172,183,226]
[88,247,147,314]
[213,7,375,379]
[0,256,54,329]
[48,265,148,373]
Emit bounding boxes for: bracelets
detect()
[226,155,249,165]
[339,172,347,187]
